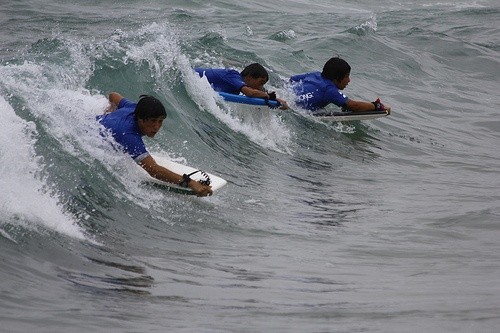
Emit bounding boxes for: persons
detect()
[289,53,391,117]
[193,63,289,110]
[95,91,213,197]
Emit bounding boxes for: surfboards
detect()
[128,156,227,196]
[315,105,392,122]
[213,92,283,112]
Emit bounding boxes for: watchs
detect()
[371,101,381,111]
